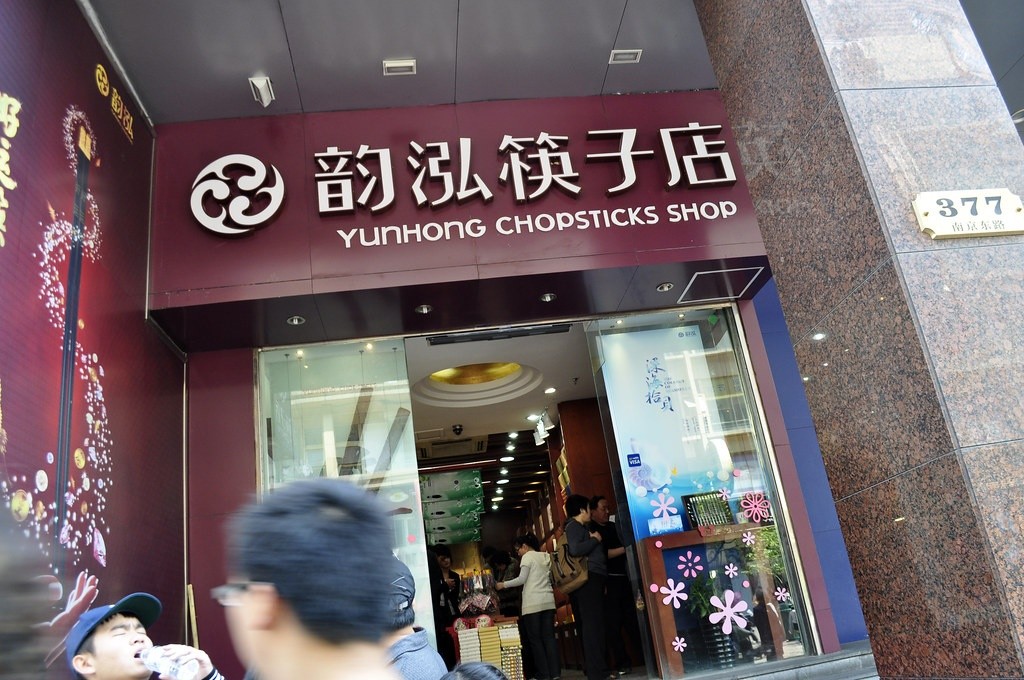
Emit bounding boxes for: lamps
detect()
[541,411,555,430]
[537,420,550,439]
[249,77,276,108]
[533,429,546,446]
[383,59,416,76]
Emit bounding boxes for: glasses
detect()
[515,546,522,553]
[209,580,276,607]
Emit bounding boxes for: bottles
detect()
[140,646,201,680]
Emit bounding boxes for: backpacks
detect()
[549,520,589,594]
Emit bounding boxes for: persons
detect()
[67,592,225,680]
[426,544,461,671]
[563,494,645,680]
[221,479,511,680]
[496,531,561,680]
[482,546,535,680]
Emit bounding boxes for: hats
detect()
[386,555,415,605]
[65,592,162,680]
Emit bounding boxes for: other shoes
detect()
[609,669,633,679]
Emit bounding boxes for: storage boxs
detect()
[458,624,524,680]
